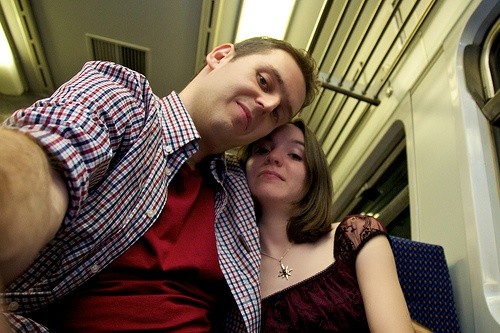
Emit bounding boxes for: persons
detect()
[237,116,415,333]
[1,35,321,332]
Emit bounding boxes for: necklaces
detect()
[261,239,297,279]
